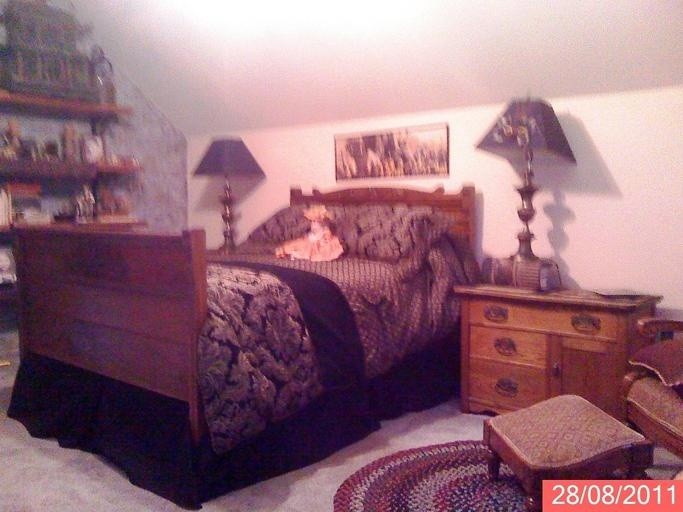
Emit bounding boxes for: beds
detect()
[7,183,474,499]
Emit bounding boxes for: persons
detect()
[0,248,17,285]
[273,202,344,264]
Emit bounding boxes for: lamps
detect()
[474,95,579,262]
[192,138,267,248]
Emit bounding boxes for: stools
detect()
[482,392,655,511]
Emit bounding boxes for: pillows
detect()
[629,332,683,387]
[238,204,458,283]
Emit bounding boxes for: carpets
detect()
[333,440,652,512]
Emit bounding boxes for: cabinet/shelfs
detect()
[0,89,150,324]
[451,285,664,430]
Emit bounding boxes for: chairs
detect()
[619,316,683,458]
